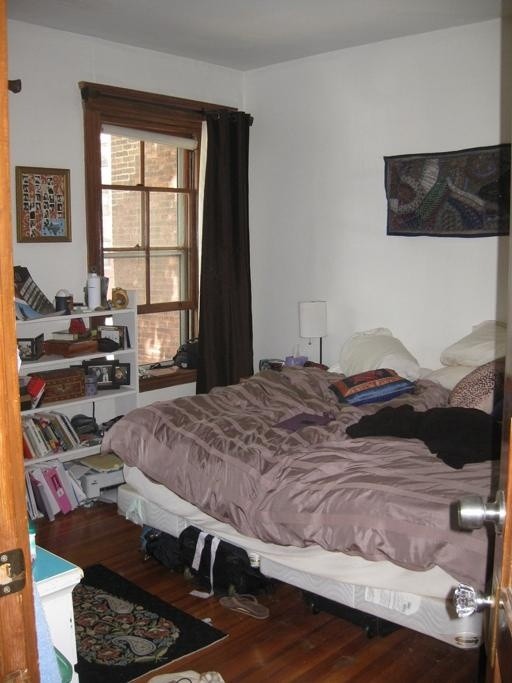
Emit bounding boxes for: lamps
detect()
[299,302,329,365]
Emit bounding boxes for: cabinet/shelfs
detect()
[17,306,142,466]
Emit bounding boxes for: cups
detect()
[55,296,71,315]
[85,375,97,396]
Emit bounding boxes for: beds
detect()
[97,370,495,650]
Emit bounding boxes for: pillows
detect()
[339,324,418,379]
[327,369,416,408]
[419,368,477,389]
[449,356,504,421]
[441,319,508,366]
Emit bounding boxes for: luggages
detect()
[302,590,406,639]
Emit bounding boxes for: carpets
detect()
[74,564,228,682]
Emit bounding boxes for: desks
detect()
[31,546,84,681]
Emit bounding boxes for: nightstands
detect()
[266,360,328,371]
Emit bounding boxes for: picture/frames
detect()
[16,166,72,243]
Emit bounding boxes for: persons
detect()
[22,175,66,237]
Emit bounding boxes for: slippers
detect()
[218,592,270,621]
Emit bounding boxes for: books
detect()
[19,410,80,460]
[24,459,88,522]
[50,328,98,341]
[78,452,124,473]
[26,376,46,409]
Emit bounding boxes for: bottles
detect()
[87,273,101,309]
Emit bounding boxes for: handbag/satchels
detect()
[174,338,199,369]
[177,525,251,600]
[145,529,183,576]
